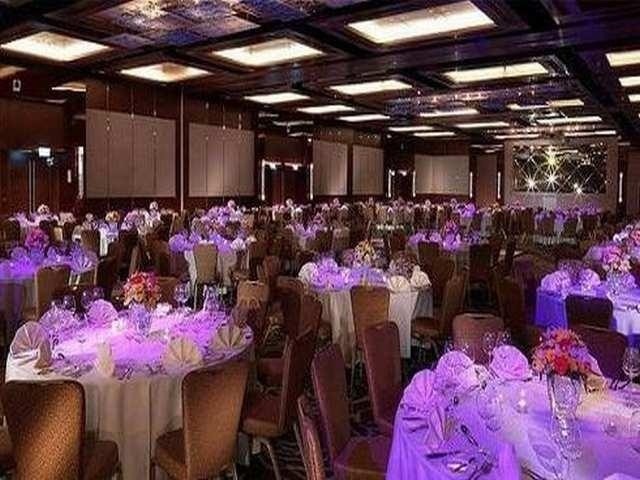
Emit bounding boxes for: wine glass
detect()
[403,328,640,480]
[19,285,222,375]
[579,273,623,301]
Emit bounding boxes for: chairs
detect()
[0,196,640,479]
